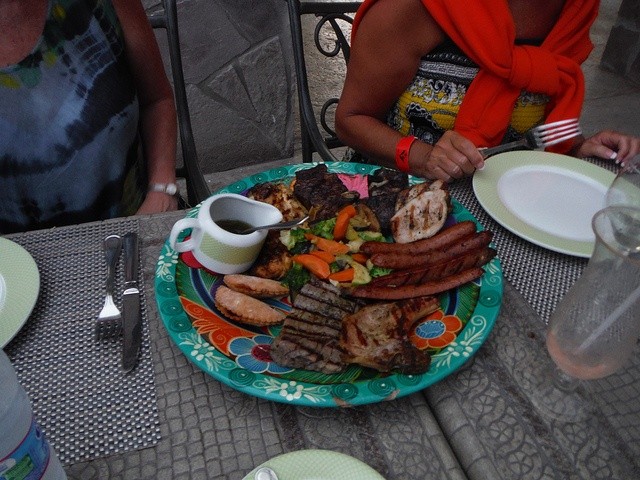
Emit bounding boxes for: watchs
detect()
[146,182,179,198]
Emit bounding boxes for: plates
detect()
[0,235,40,352]
[472,150,640,259]
[240,448,386,480]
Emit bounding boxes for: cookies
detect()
[215,286,286,328]
[223,274,289,297]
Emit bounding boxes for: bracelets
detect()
[395,135,418,173]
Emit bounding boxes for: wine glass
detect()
[523,206,640,425]
[601,151,640,266]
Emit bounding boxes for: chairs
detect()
[145,0,211,209]
[289,0,363,162]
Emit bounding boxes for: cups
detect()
[167,192,284,276]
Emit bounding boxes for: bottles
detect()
[0,351,68,480]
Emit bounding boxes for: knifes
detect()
[119,231,144,375]
[152,162,504,408]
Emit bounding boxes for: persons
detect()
[335,0,640,183]
[0,0,180,235]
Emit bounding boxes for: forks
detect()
[478,117,582,158]
[94,235,123,342]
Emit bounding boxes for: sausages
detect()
[352,220,497,299]
